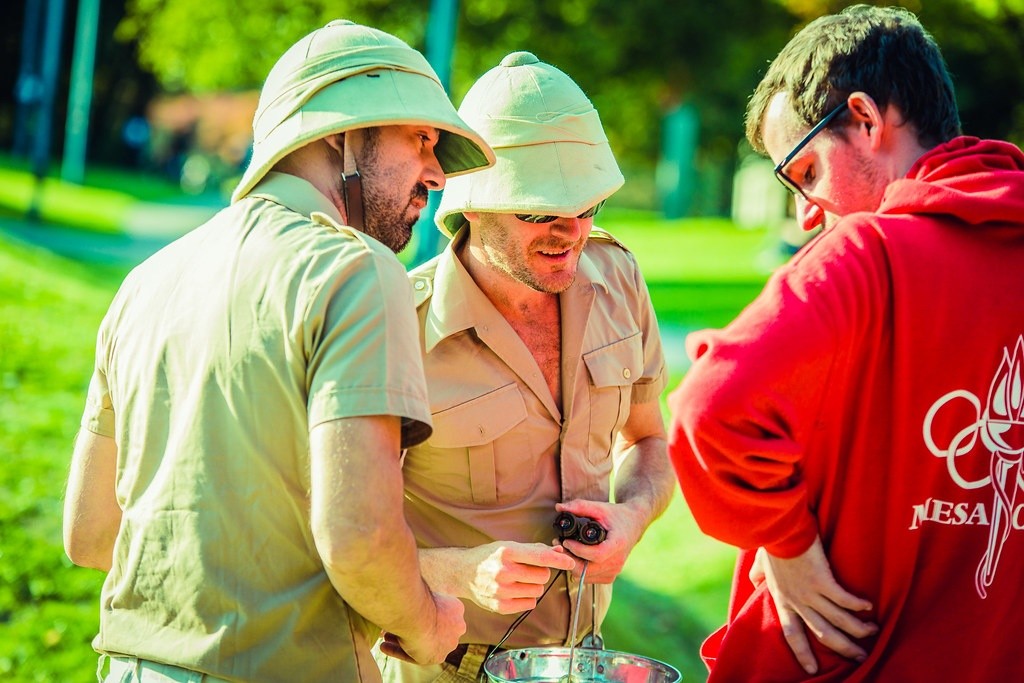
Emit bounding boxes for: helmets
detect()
[230,17,495,200]
[433,50,627,243]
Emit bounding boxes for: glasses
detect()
[513,197,606,223]
[773,98,887,200]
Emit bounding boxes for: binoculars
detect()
[553,509,607,545]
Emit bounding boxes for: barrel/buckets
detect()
[484,646,683,683]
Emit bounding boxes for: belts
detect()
[445,644,508,674]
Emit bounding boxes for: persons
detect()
[667,4,1024,683]
[61,19,683,683]
